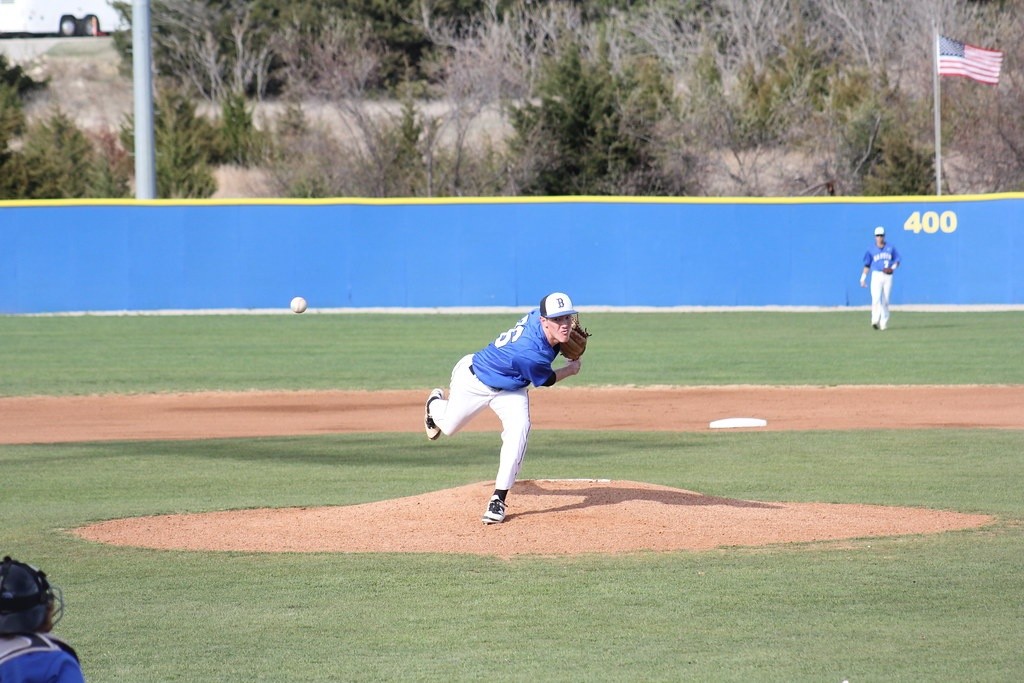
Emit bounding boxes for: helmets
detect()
[0,556,65,635]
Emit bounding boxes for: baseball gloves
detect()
[560,313,589,361]
[884,267,893,274]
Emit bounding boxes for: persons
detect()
[425,292,581,523]
[0,556,85,683]
[860,226,901,330]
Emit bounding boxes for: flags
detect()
[937,34,1003,85]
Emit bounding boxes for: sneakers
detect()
[482,495,508,524]
[424,388,445,440]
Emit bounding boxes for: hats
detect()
[539,292,579,318]
[874,226,885,236]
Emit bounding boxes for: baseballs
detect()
[290,295,307,313]
